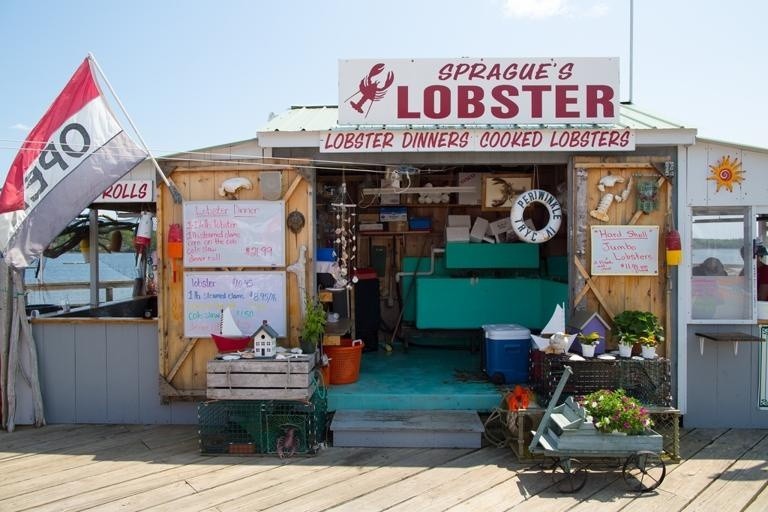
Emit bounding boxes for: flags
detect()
[1,56,149,274]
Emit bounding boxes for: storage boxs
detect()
[484,323,533,387]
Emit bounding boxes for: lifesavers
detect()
[510,190,562,243]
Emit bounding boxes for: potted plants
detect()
[641,332,659,359]
[620,335,633,358]
[579,330,602,362]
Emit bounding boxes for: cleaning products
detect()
[317,352,334,389]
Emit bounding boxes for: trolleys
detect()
[528,364,666,493]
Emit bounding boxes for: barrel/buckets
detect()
[323,339,366,383]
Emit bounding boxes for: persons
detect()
[736,244,768,301]
[694,258,729,321]
